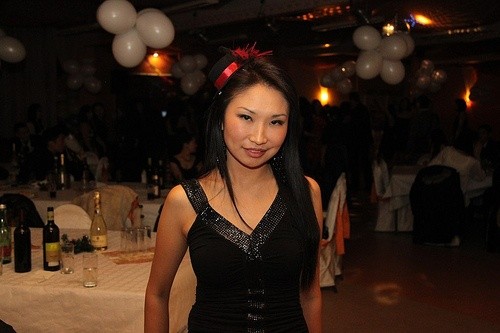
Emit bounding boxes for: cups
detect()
[121,226,151,248]
[82,253,98,288]
[90,192,107,252]
[61,243,74,274]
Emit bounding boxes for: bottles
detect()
[0,204,11,264]
[14,209,31,273]
[147,158,161,198]
[52,153,94,197]
[43,207,60,271]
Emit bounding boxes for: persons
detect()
[8,92,500,199]
[144,53,322,333]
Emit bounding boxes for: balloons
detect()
[0,36,27,63]
[96,0,174,68]
[352,26,416,85]
[416,56,448,89]
[65,61,101,91]
[173,55,209,95]
[320,60,357,94]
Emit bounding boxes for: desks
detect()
[0,178,222,333]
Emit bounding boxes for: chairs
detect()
[45,204,93,229]
[319,171,349,292]
[372,159,414,234]
